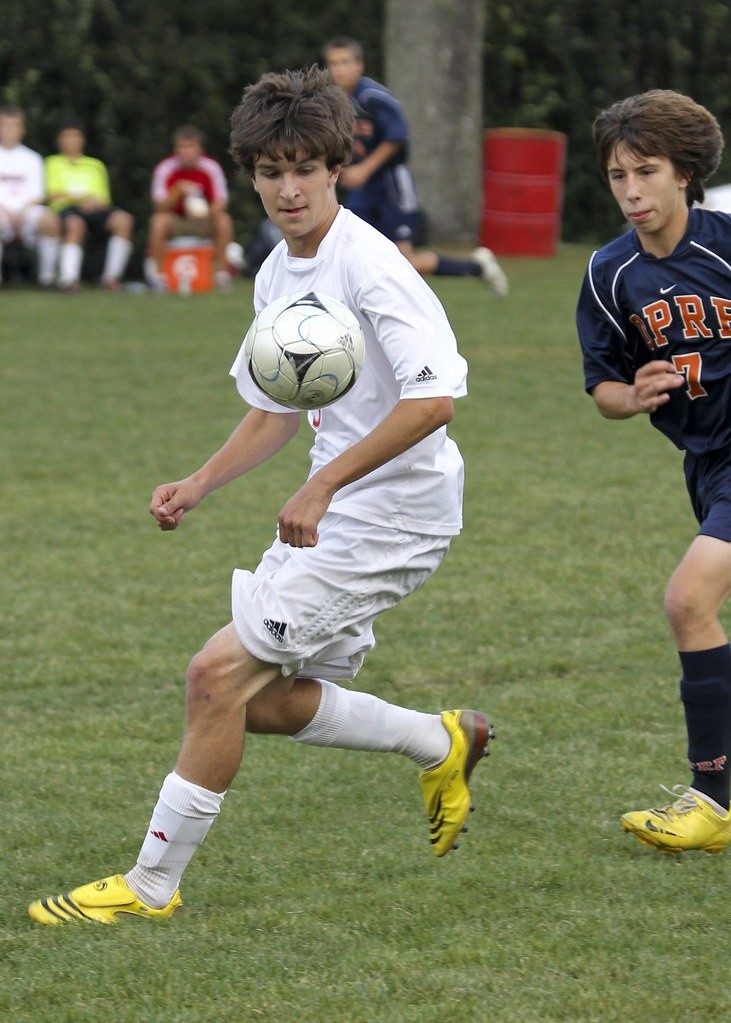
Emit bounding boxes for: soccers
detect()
[244,291,365,411]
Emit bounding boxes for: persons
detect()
[33,111,139,292]
[0,105,60,288]
[568,89,731,855]
[139,123,243,291]
[23,61,494,928]
[312,37,508,296]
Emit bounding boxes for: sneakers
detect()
[420,708,495,857]
[470,247,509,296]
[619,790,730,854]
[27,873,181,926]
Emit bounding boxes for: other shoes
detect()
[36,274,233,294]
